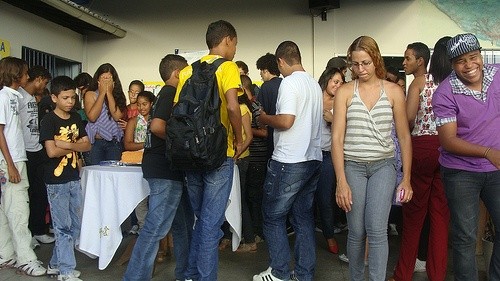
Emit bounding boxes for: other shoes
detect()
[315,224,341,232]
[413,258,427,272]
[327,239,339,254]
[254,234,265,243]
[219,238,232,250]
[388,223,399,235]
[156,249,167,262]
[48,224,56,234]
[287,226,296,235]
[339,253,350,263]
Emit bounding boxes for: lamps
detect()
[310,0,340,20]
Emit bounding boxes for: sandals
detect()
[237,243,258,253]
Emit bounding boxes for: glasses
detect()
[351,59,373,68]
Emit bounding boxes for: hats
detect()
[448,33,482,61]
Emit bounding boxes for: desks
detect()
[74,161,242,270]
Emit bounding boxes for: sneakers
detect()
[59,274,82,281]
[30,237,41,249]
[46,264,81,279]
[129,225,141,236]
[35,234,56,244]
[254,266,287,281]
[289,270,299,281]
[0,257,17,269]
[16,260,47,277]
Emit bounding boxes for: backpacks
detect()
[164,60,228,175]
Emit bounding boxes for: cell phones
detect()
[400,189,405,200]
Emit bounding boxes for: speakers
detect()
[309,0,340,11]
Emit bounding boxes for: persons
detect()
[221,33,500,281]
[0,20,244,281]
